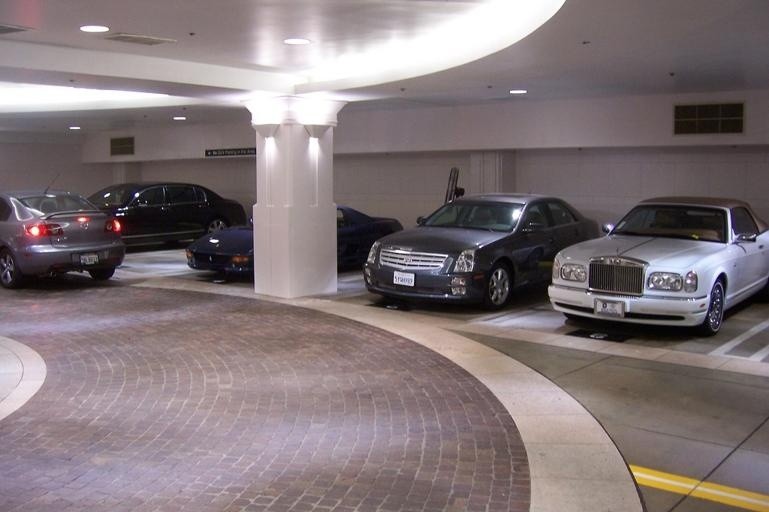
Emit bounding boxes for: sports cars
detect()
[179,202,407,278]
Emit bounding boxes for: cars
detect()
[358,191,600,315]
[79,176,247,257]
[0,171,127,292]
[545,192,769,339]
